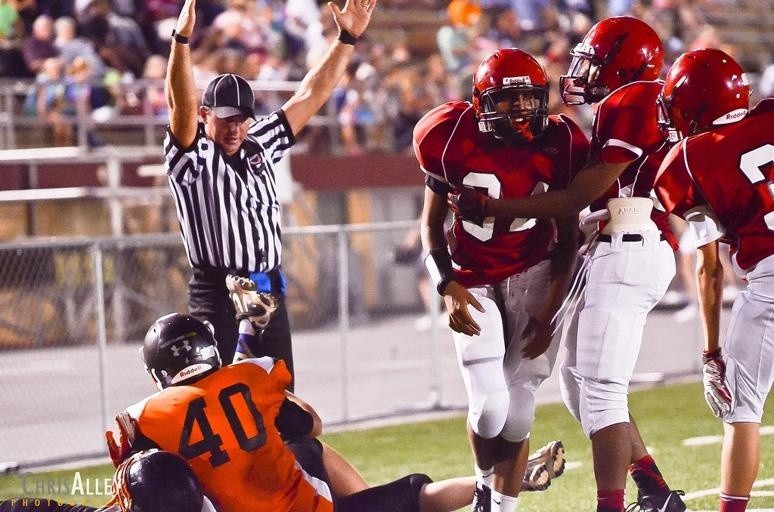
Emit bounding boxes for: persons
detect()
[2,0,774,158]
[412,44,593,509]
[0,447,218,512]
[104,310,566,512]
[444,13,690,510]
[221,269,279,366]
[665,210,744,324]
[159,1,377,402]
[651,47,774,510]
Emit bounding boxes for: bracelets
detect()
[420,245,454,293]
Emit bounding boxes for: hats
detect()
[202,74,257,121]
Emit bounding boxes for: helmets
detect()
[472,17,750,141]
[138,313,223,390]
[110,448,203,512]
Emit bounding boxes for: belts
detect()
[598,234,666,242]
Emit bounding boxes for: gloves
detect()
[447,183,490,228]
[703,347,732,417]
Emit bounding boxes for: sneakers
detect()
[225,274,278,333]
[625,490,686,512]
[472,481,491,512]
[521,440,565,491]
